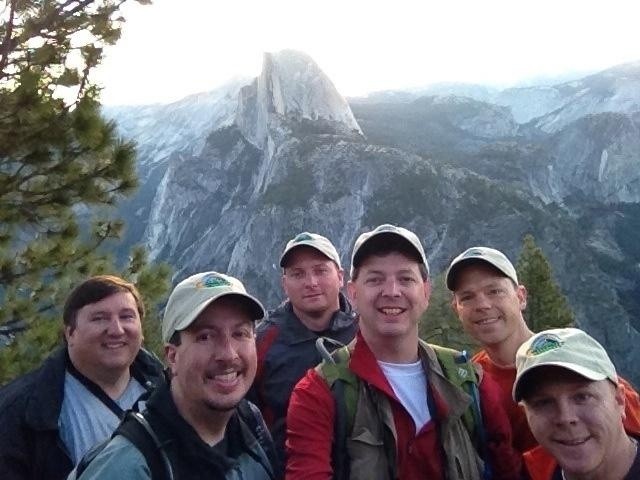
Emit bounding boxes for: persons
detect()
[512,328,640,480]
[0,276,164,480]
[284,223,483,480]
[445,246,640,479]
[247,232,361,479]
[67,271,279,480]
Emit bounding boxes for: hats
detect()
[445,247,518,291]
[512,328,618,404]
[280,232,340,267]
[350,224,429,276]
[162,271,264,342]
